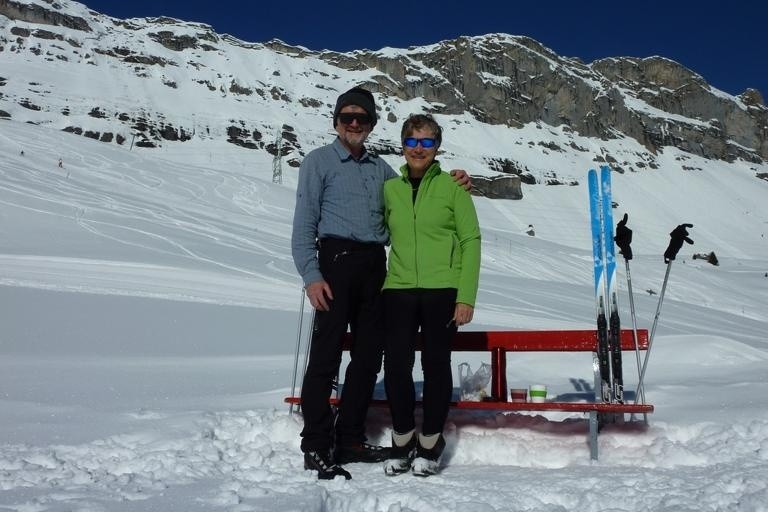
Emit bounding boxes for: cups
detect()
[529,384,547,403]
[510,388,528,403]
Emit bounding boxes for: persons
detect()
[382,115,483,476]
[290,86,473,481]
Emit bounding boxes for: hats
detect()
[333,89,376,131]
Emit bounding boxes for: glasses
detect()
[404,137,435,147]
[339,112,370,125]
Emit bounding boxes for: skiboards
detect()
[588,165,626,427]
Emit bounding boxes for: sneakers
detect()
[332,444,392,464]
[383,433,417,475]
[411,433,446,477]
[304,449,352,480]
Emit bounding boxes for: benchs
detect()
[281,326,655,464]
